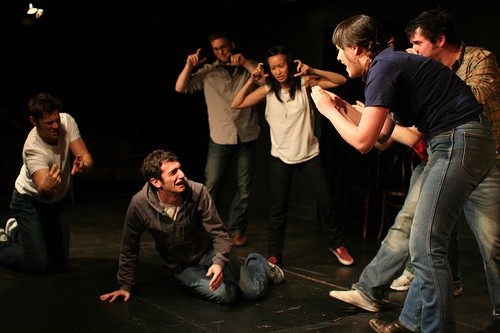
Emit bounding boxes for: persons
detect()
[0,92,95,290]
[174,31,266,247]
[230,45,354,266]
[310,8,500,333]
[99,148,285,309]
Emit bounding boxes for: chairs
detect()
[342,153,414,240]
